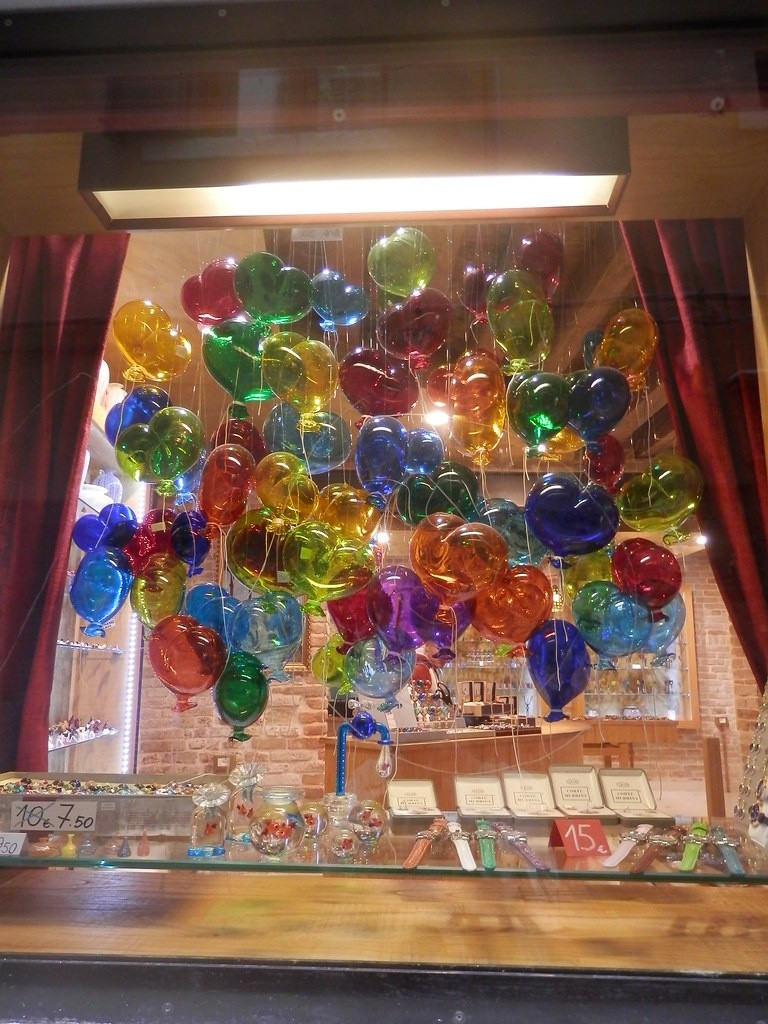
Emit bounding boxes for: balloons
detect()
[475,565,552,643]
[485,262,553,367]
[68,227,706,743]
[237,252,311,332]
[111,299,193,392]
[366,227,435,296]
[357,414,444,510]
[527,469,620,552]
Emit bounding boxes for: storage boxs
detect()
[385,765,675,829]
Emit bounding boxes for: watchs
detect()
[494,820,551,870]
[477,816,497,871]
[601,824,654,870]
[678,822,710,873]
[446,821,476,872]
[631,824,680,878]
[403,818,447,870]
[711,826,745,875]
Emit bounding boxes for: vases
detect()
[104,382,128,413]
[80,468,123,512]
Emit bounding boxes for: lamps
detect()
[76,115,632,230]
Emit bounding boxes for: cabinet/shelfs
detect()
[320,727,589,812]
[47,499,123,752]
[455,644,681,746]
